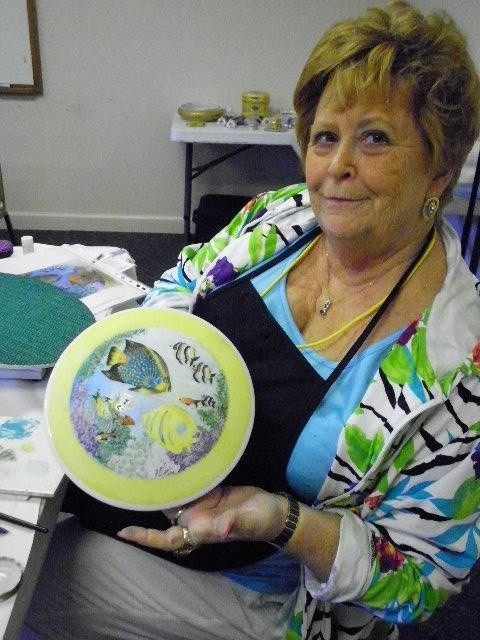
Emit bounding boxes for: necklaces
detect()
[319,238,399,318]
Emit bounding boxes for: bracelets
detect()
[268,489,300,547]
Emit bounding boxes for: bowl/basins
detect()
[177,102,226,123]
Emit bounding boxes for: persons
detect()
[17,0,480,640]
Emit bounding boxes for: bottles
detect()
[20,235,35,255]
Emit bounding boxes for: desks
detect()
[0,246,129,640]
[171,116,316,247]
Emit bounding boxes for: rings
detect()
[170,508,186,525]
[173,524,196,561]
